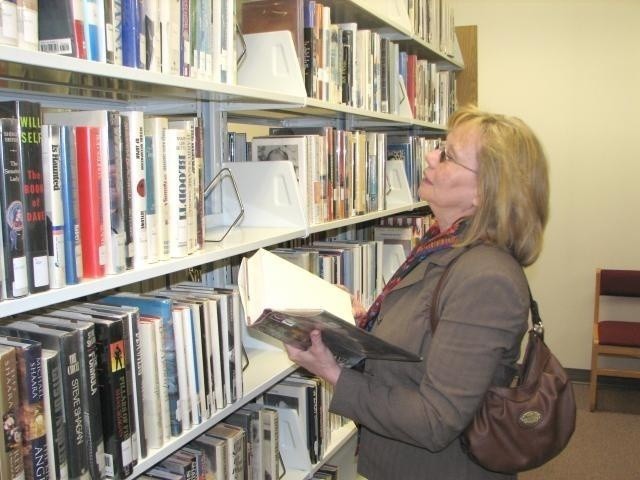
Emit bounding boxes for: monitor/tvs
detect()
[0,0,240,86]
[237,248,424,369]
[305,0,466,126]
[268,214,434,310]
[243,373,352,479]
[233,125,445,223]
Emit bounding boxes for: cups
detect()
[435,141,478,175]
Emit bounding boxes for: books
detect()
[233,125,445,223]
[268,214,434,310]
[0,282,244,479]
[0,99,206,299]
[305,0,466,126]
[243,373,352,479]
[237,248,424,369]
[0,0,240,86]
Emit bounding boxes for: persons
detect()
[284,106,550,479]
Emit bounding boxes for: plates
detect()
[1,0,480,480]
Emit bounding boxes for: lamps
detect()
[589,269,640,412]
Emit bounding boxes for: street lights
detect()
[431,244,576,473]
